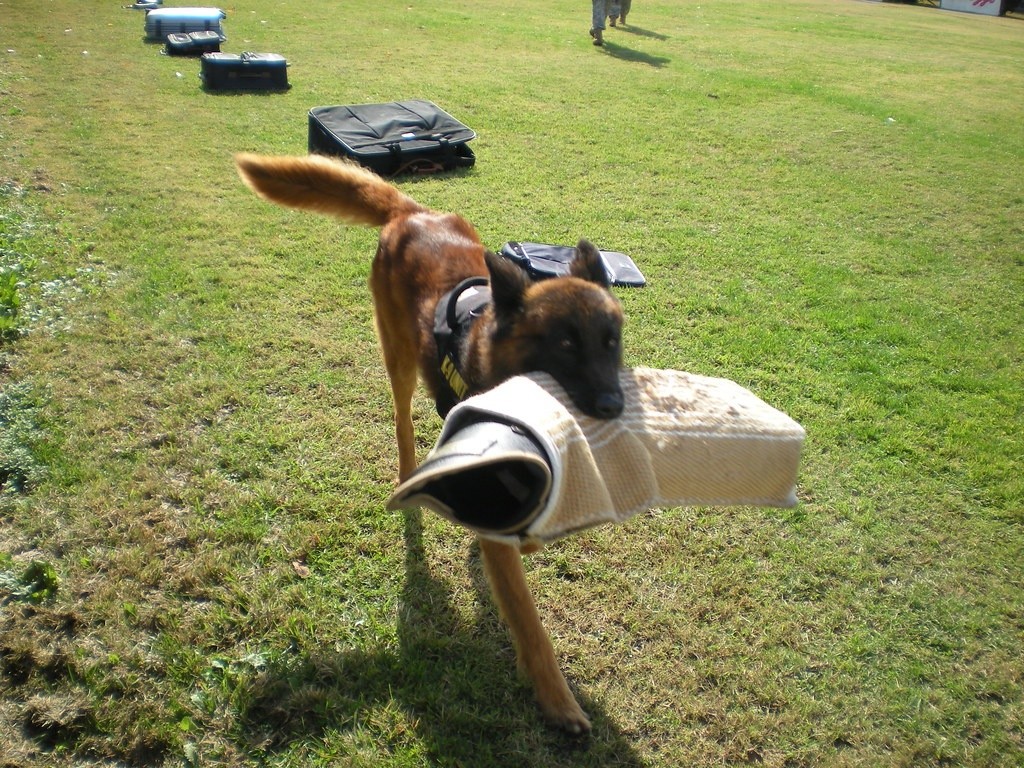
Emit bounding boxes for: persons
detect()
[589,0,632,46]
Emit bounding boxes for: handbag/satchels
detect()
[308,99,478,182]
[500,239,647,288]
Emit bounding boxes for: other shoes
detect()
[618,16,626,25]
[589,26,603,45]
[609,15,617,27]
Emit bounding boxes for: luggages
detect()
[198,48,292,93]
[143,8,226,45]
[159,30,221,58]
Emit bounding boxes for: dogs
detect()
[233,152,623,736]
